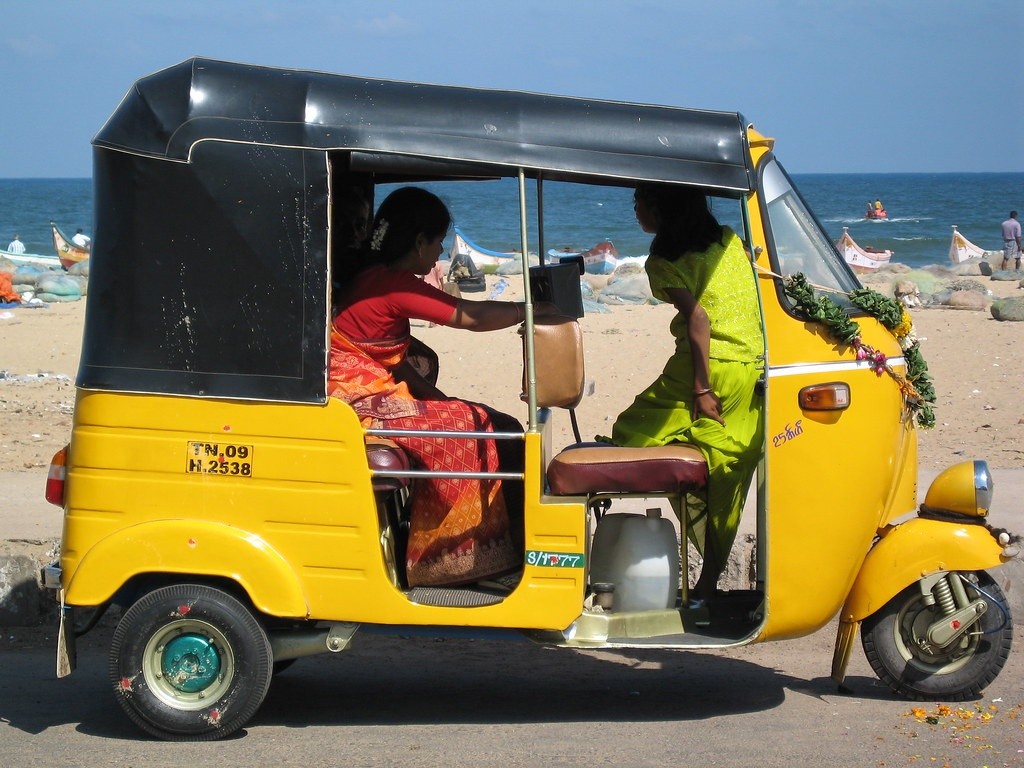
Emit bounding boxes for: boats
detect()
[832,226,896,275]
[547,237,618,275]
[449,223,519,273]
[48,221,90,268]
[866,209,888,219]
[950,225,1024,269]
[1,250,64,272]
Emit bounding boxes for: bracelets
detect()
[694,387,713,395]
[510,301,521,325]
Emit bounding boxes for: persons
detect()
[875,199,881,209]
[71,228,91,247]
[867,200,872,210]
[1001,211,1023,271]
[595,188,766,603]
[8,234,26,254]
[329,186,561,588]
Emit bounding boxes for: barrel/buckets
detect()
[591,506,680,609]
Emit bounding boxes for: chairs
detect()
[363,436,412,590]
[518,315,710,609]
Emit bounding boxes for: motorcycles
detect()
[42,53,1012,737]
